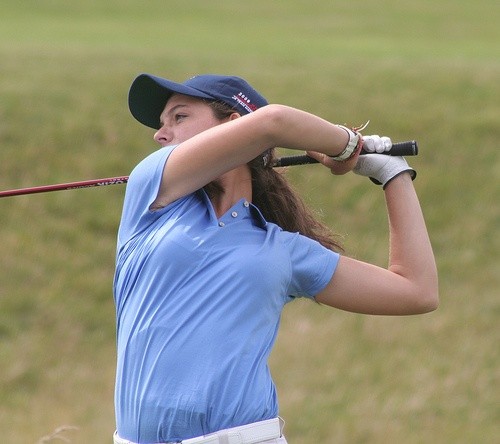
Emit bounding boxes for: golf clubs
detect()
[0,139,418,197]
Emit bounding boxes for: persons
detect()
[114,75,440,444]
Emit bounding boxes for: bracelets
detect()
[327,125,360,162]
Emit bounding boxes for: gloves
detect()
[354,135,416,187]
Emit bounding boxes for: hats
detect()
[128,74,269,116]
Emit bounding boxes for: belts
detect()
[113,417,280,444]
